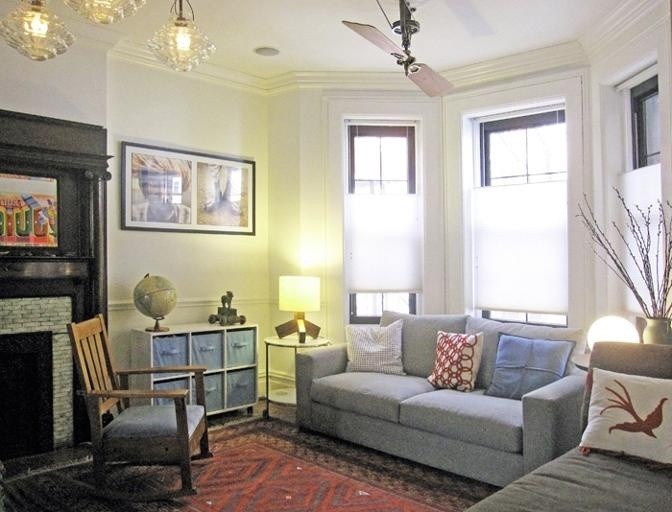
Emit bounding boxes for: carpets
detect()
[0,418,503,511]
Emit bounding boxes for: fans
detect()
[342,0,456,100]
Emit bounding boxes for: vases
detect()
[642,317,672,349]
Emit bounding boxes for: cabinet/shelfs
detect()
[130,320,261,417]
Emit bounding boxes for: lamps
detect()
[586,312,641,353]
[0,0,218,74]
[273,275,321,342]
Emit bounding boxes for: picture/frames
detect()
[0,168,64,251]
[119,140,256,238]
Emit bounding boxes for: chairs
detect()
[50,310,215,502]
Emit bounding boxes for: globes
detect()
[133,273,176,332]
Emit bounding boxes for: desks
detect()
[261,336,329,420]
[570,351,594,437]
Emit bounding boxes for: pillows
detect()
[481,332,576,400]
[342,318,409,376]
[579,367,672,472]
[427,324,484,392]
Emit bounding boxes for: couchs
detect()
[290,310,589,489]
[447,340,671,512]
